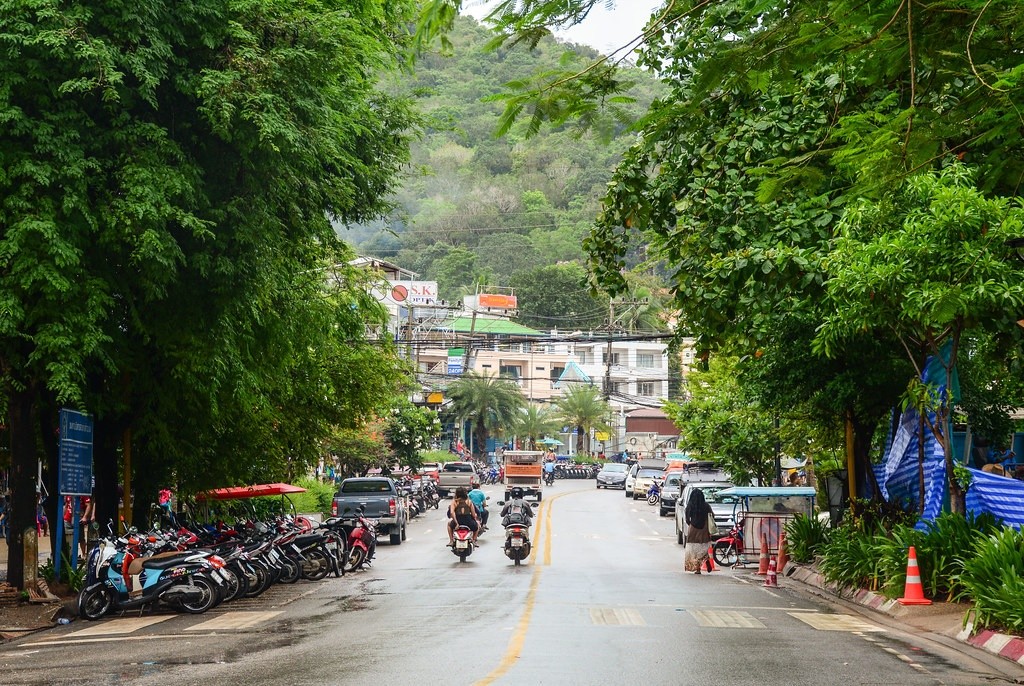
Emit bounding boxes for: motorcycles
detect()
[646,479,666,505]
[544,462,602,487]
[712,486,816,567]
[500,450,544,501]
[447,496,539,567]
[474,465,504,485]
[393,478,441,519]
[76,505,389,620]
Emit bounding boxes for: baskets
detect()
[361,530,378,547]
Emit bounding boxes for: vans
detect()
[678,465,732,495]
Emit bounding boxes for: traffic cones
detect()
[897,545,933,606]
[755,533,785,588]
[701,547,720,571]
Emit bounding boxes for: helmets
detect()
[511,487,523,498]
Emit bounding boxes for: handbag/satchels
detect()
[707,507,718,534]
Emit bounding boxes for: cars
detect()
[407,462,442,482]
[659,471,683,517]
[674,481,748,547]
[597,463,670,501]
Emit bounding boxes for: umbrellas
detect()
[536,439,564,454]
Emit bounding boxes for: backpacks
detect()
[509,498,528,524]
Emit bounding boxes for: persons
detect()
[684,488,716,574]
[985,441,1017,473]
[444,436,644,547]
[0,477,171,564]
[785,472,806,488]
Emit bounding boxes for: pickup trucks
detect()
[330,477,407,546]
[438,461,482,498]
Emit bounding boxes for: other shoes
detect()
[695,572,701,574]
[705,561,712,573]
[479,527,487,532]
[446,541,452,546]
[483,524,489,530]
[472,539,479,547]
